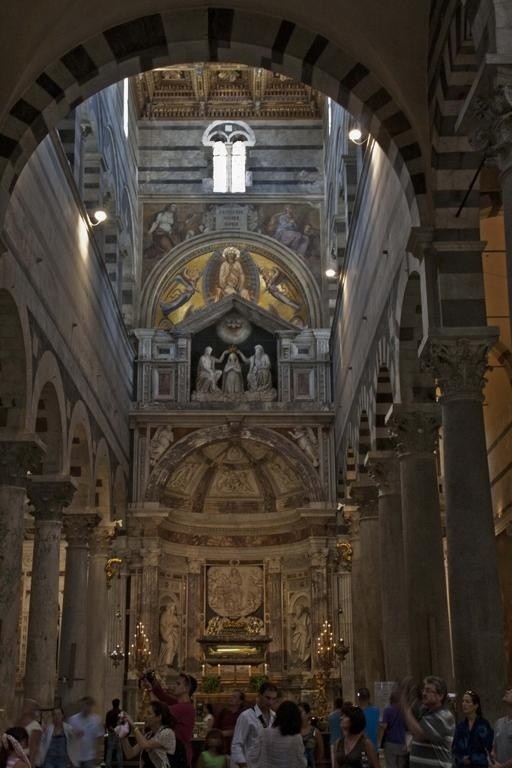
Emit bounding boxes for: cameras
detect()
[117,712,124,720]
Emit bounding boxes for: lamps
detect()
[91,207,107,227]
[110,558,127,669]
[333,574,350,665]
[348,127,370,146]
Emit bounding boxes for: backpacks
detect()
[159,727,187,768]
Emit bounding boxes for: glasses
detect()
[465,690,475,696]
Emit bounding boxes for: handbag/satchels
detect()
[359,750,380,768]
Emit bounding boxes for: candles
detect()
[200,662,205,678]
[262,661,269,675]
[247,663,253,678]
[217,663,221,678]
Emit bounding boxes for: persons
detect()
[197,347,229,392]
[159,602,181,666]
[146,204,179,256]
[288,604,313,660]
[288,425,319,467]
[153,425,175,455]
[221,353,244,393]
[218,249,249,302]
[273,205,310,255]
[226,569,242,610]
[235,344,272,392]
